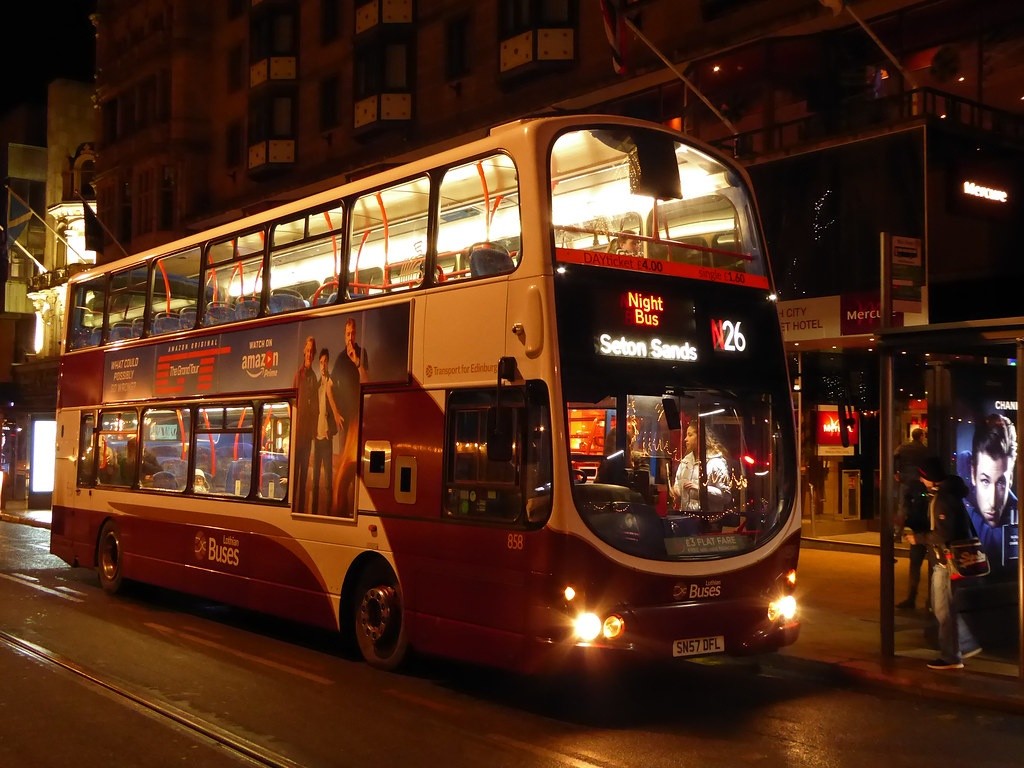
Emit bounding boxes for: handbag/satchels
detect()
[932,563,952,624]
[944,542,991,579]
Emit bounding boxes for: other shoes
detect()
[959,645,983,658]
[896,600,915,609]
[927,659,966,668]
[894,534,901,542]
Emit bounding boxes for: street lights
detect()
[13,425,22,500]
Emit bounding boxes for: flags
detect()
[600,0,626,74]
[83,201,104,256]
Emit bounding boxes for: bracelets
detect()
[356,357,361,368]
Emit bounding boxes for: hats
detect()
[917,458,946,481]
[195,468,206,484]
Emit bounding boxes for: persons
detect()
[594,428,634,487]
[962,414,1019,583]
[84,433,119,484]
[605,229,639,257]
[895,473,928,609]
[892,429,928,543]
[326,318,369,518]
[901,458,982,669]
[312,348,339,516]
[184,469,210,493]
[270,436,289,498]
[669,420,732,512]
[120,436,164,487]
[293,336,317,513]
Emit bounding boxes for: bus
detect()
[49,112,805,691]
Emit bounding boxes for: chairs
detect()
[647,229,746,271]
[142,443,292,498]
[90,241,516,347]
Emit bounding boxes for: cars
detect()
[218,443,284,463]
[148,445,184,464]
